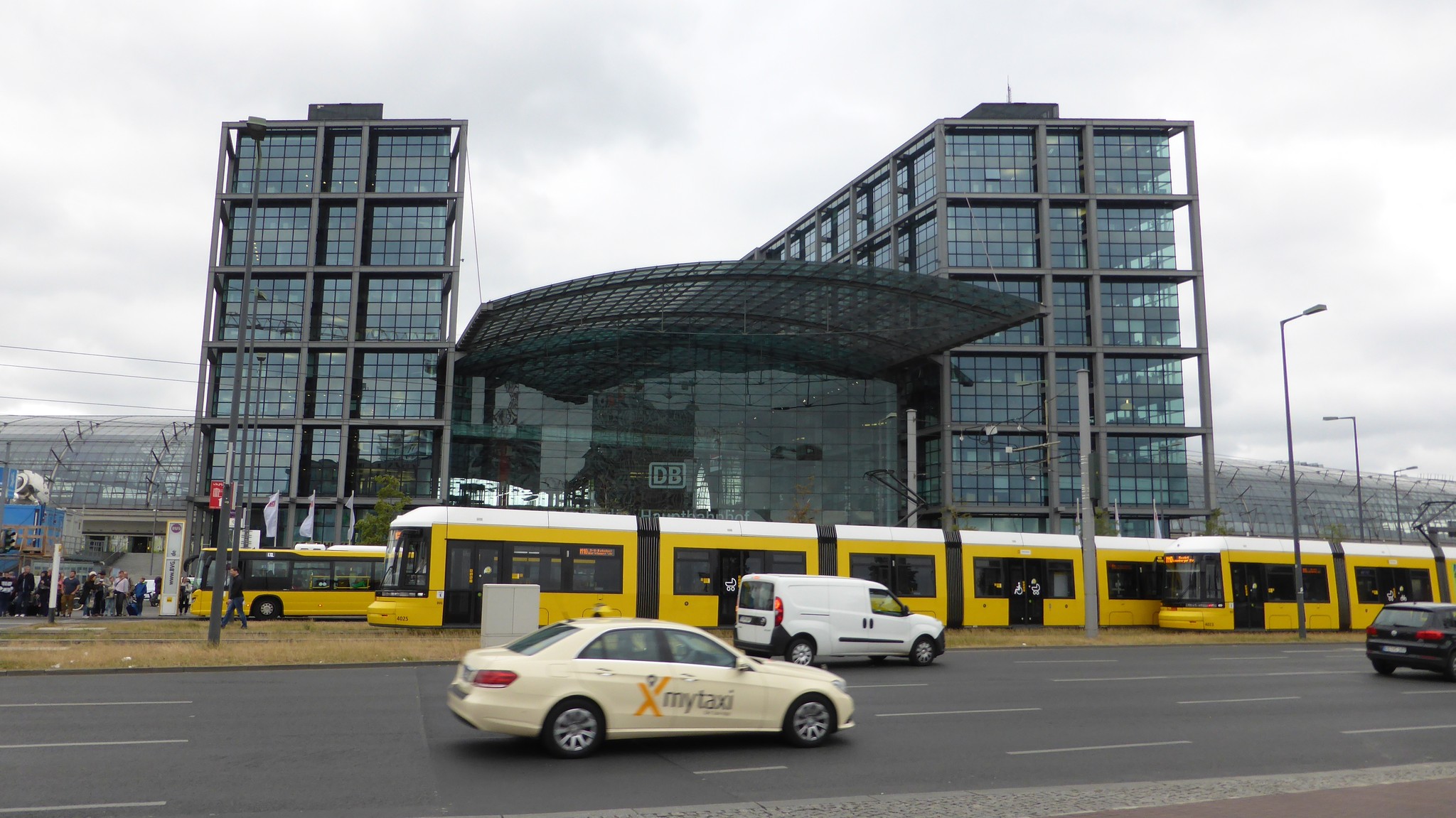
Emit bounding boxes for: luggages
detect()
[125,596,139,616]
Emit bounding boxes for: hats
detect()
[98,570,106,575]
[89,571,97,576]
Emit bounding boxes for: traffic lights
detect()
[4,529,16,553]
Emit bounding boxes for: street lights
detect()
[1158,442,1183,539]
[226,287,267,623]
[1016,379,1054,534]
[1394,466,1418,544]
[1280,304,1328,638]
[878,412,897,527]
[207,116,269,647]
[244,356,267,550]
[1323,417,1364,543]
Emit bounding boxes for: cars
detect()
[445,618,856,760]
[1366,601,1456,682]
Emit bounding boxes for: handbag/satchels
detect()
[106,592,110,596]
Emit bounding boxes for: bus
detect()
[734,576,947,670]
[1151,535,1455,633]
[367,506,1176,630]
[184,543,596,621]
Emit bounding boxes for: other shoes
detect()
[36,614,46,617]
[241,626,247,629]
[221,626,225,629]
[61,614,65,617]
[179,613,183,616]
[66,614,71,618]
[184,612,186,615]
[55,613,60,616]
[14,613,20,617]
[137,613,141,616]
[2,611,11,617]
[19,613,25,617]
[82,614,122,619]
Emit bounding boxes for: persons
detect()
[77,570,135,619]
[0,566,67,618]
[129,577,147,617]
[60,570,81,618]
[179,577,193,616]
[220,566,247,629]
[255,566,381,590]
[646,636,702,664]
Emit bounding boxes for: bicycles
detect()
[66,591,84,611]
